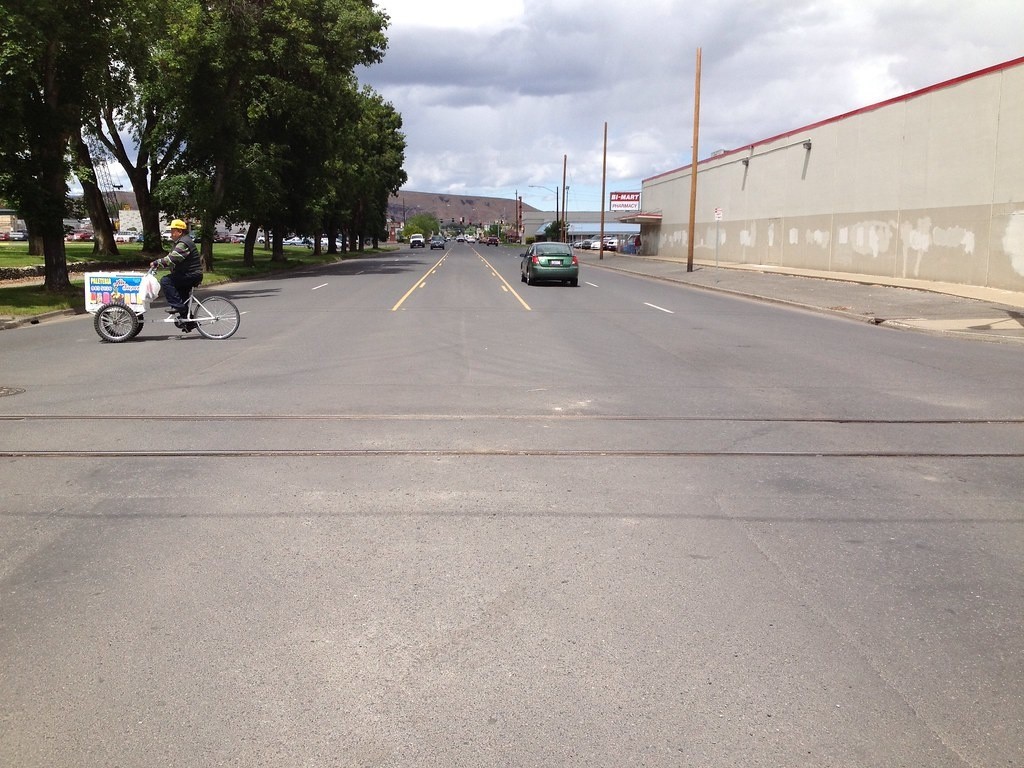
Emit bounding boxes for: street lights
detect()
[565,185,570,242]
[529,184,559,241]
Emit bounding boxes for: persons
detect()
[148,218,206,335]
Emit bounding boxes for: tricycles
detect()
[83,260,239,342]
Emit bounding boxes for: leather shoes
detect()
[182,323,200,332]
[165,306,186,313]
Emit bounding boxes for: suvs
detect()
[430,235,445,250]
[410,233,425,248]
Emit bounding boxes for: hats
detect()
[165,219,187,229]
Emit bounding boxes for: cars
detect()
[0,229,361,252]
[519,241,579,286]
[446,234,499,246]
[571,232,640,256]
